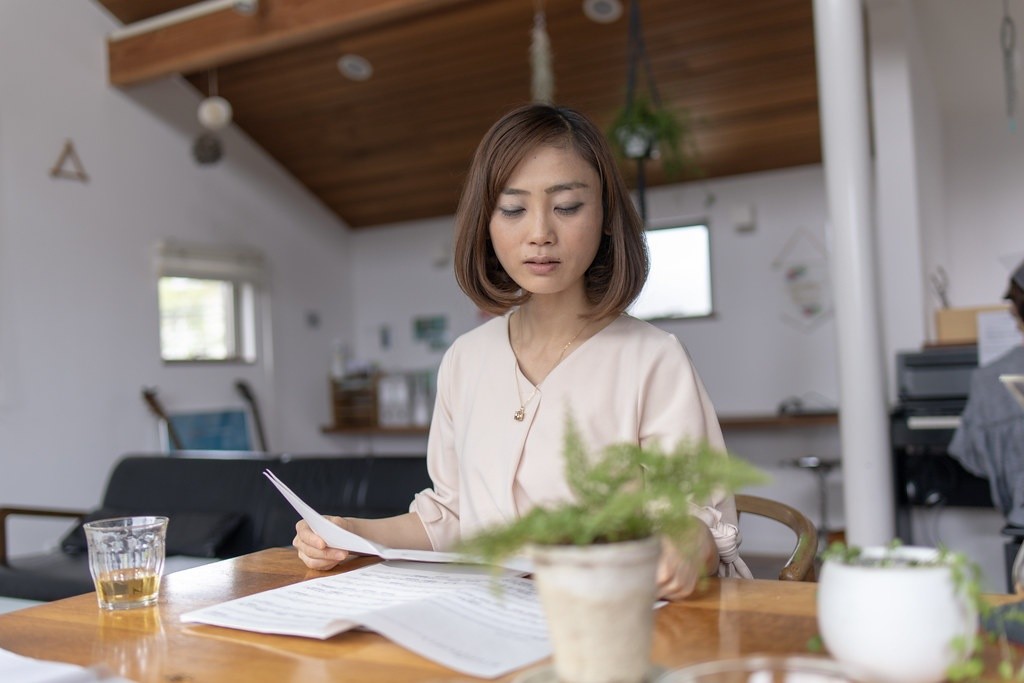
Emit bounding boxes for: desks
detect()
[321,411,840,436]
[0,543,1024,683]
[103,450,435,559]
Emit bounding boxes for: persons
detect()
[948,263,1024,594]
[291,100,753,603]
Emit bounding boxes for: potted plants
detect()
[810,535,1024,683]
[609,96,717,206]
[458,413,770,683]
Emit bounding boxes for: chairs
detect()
[735,489,817,581]
[0,504,250,602]
[140,382,270,451]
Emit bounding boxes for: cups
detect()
[652,654,869,683]
[84,516,169,609]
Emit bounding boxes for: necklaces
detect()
[510,308,587,421]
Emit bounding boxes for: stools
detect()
[780,457,844,534]
